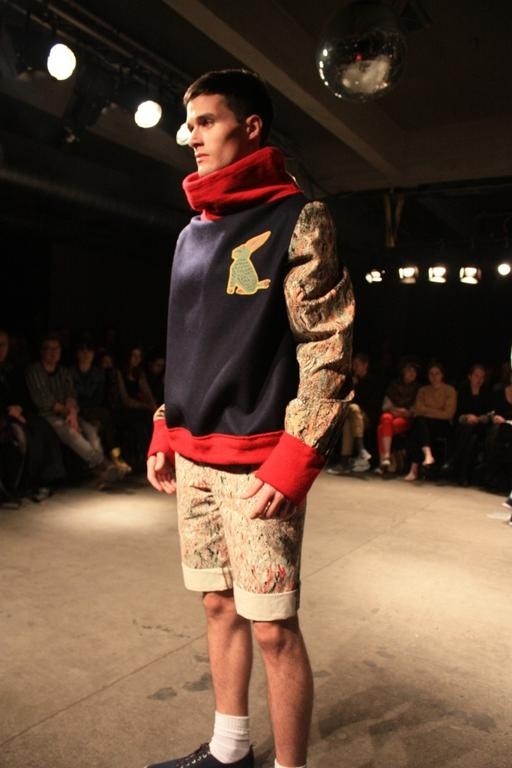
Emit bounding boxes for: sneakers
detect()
[1,462,132,508]
[325,451,466,485]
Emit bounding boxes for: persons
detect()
[326,353,512,501]
[0,332,168,509]
[143,66,356,768]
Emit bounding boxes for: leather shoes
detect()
[143,742,255,768]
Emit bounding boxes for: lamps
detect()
[160,101,194,146]
[16,0,77,83]
[117,80,169,130]
[366,236,512,285]
